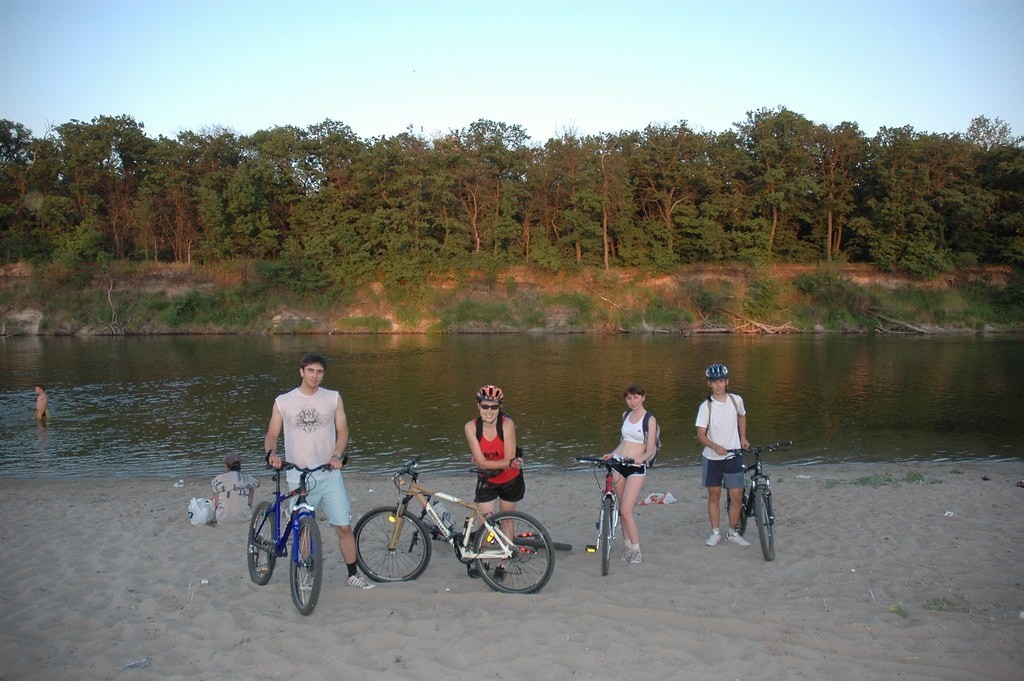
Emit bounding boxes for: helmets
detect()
[705,363,728,380]
[476,385,504,404]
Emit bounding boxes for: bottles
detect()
[432,501,456,529]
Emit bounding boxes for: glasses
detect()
[480,403,499,410]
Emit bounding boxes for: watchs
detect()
[331,454,343,460]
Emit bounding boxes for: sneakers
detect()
[726,533,752,546]
[347,573,377,590]
[705,533,722,546]
[493,564,505,583]
[620,546,632,561]
[472,562,490,579]
[631,548,642,563]
[299,576,313,591]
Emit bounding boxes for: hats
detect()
[224,453,240,466]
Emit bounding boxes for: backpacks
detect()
[621,406,662,468]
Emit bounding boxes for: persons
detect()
[464,385,525,581]
[211,453,259,522]
[35,385,48,419]
[695,364,750,546]
[264,353,376,590]
[603,385,656,564]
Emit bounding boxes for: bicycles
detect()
[354,455,556,594]
[576,456,645,576]
[408,494,572,554]
[247,449,348,615]
[725,441,793,561]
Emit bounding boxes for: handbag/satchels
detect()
[188,497,217,526]
